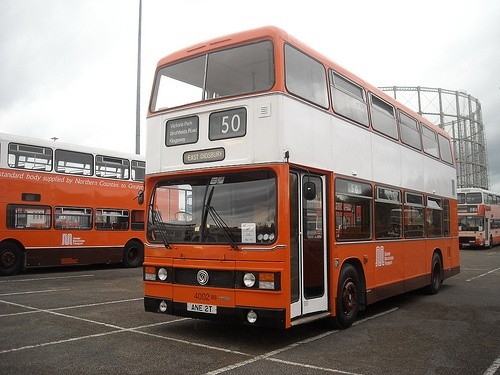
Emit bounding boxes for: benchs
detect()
[337,224,448,239]
[96,223,144,230]
[10,161,117,179]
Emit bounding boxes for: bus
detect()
[456,187,500,249]
[0,131,178,274]
[136,23,457,331]
